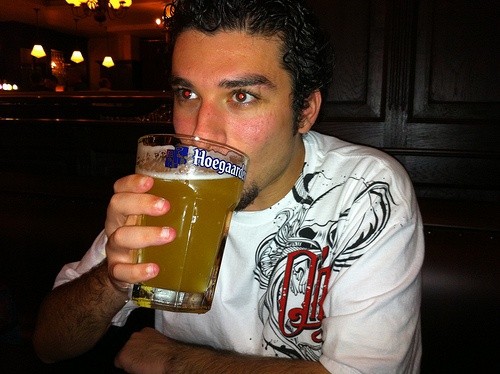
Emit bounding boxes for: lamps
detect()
[66,0,133,25]
[101,26,116,69]
[69,17,84,64]
[28,8,47,58]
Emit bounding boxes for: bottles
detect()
[50,53,64,84]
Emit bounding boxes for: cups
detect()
[130,134,248,313]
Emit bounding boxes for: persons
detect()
[26,0,425,374]
